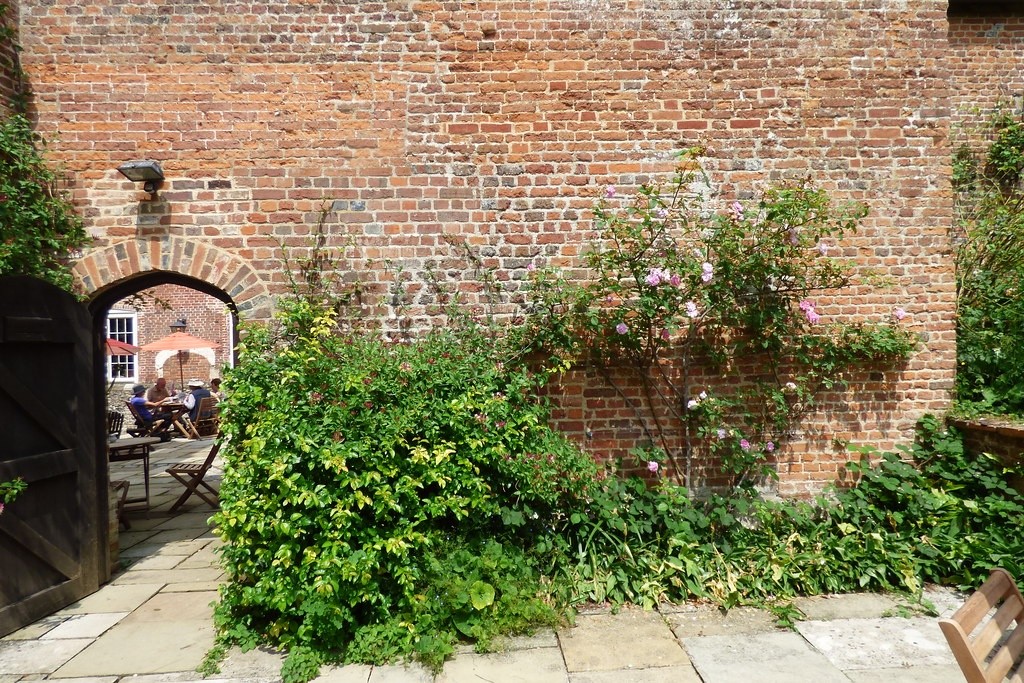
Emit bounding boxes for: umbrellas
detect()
[107,338,142,356]
[142,331,222,392]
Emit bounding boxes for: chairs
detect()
[108,411,124,456]
[125,400,164,437]
[165,430,224,514]
[188,397,220,441]
[111,479,132,530]
[938,567,1024,683]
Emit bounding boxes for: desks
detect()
[109,437,161,521]
[161,402,191,438]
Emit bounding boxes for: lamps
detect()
[115,160,166,195]
[169,319,186,332]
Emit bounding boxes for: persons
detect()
[146,378,176,413]
[210,378,222,393]
[177,379,210,437]
[129,385,173,442]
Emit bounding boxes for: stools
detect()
[126,428,155,454]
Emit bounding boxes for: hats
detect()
[187,378,205,388]
[130,384,148,395]
